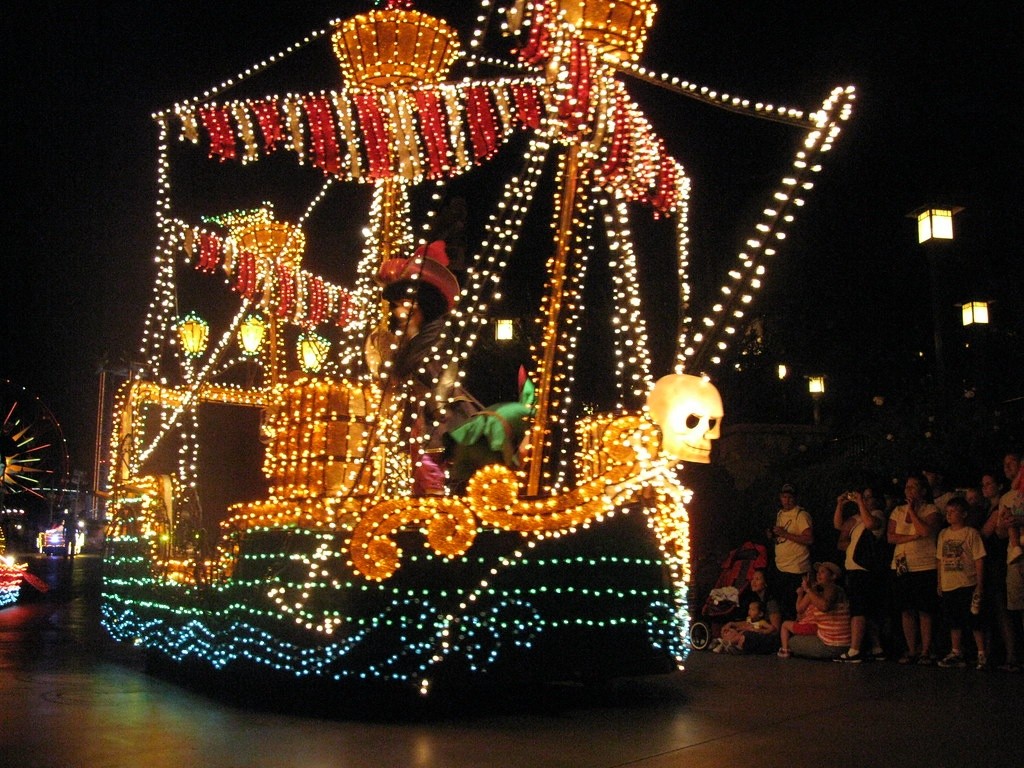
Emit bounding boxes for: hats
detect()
[814,561,843,580]
[778,481,799,498]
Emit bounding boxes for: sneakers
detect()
[833,651,864,663]
[937,651,966,668]
[974,655,989,671]
[863,650,886,661]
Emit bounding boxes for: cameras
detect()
[847,492,855,500]
[768,528,779,537]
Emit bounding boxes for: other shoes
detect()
[898,654,918,664]
[777,648,790,657]
[918,655,933,665]
[712,647,720,654]
[996,663,1019,673]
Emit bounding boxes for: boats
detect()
[86,1,857,724]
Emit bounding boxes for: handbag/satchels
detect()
[894,553,908,578]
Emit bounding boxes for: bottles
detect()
[970,593,980,614]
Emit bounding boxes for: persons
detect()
[371,279,476,498]
[713,453,1024,671]
[63,513,83,559]
